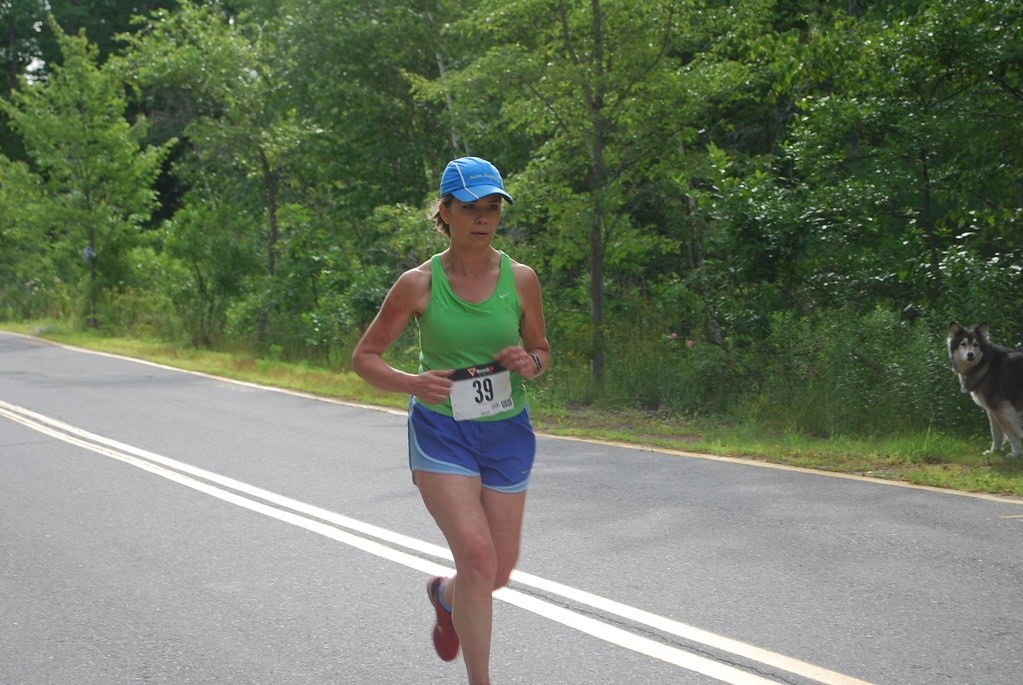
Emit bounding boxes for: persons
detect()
[351,155,550,685]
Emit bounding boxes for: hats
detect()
[440,156,514,205]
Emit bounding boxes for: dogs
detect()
[946,318,1023,460]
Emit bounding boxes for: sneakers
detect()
[427,576,459,661]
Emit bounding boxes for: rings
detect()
[520,360,525,367]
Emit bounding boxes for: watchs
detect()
[524,353,546,381]
